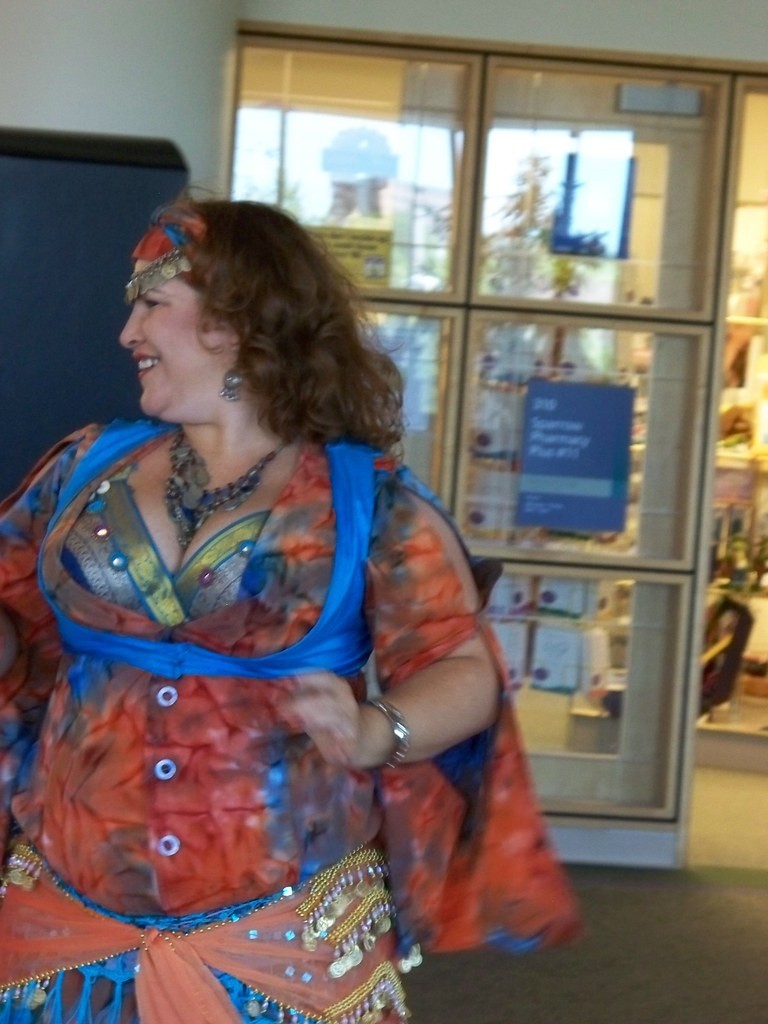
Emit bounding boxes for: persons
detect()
[1,199,581,1024]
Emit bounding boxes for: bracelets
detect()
[369,697,409,767]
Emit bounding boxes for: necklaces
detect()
[162,436,290,545]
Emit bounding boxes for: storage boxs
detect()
[470,384,522,460]
[482,568,606,696]
[468,471,519,532]
[515,378,638,529]
[311,228,397,286]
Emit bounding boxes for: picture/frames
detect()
[613,82,703,119]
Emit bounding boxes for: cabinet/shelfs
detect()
[235,31,733,824]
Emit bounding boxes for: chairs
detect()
[607,598,755,721]
